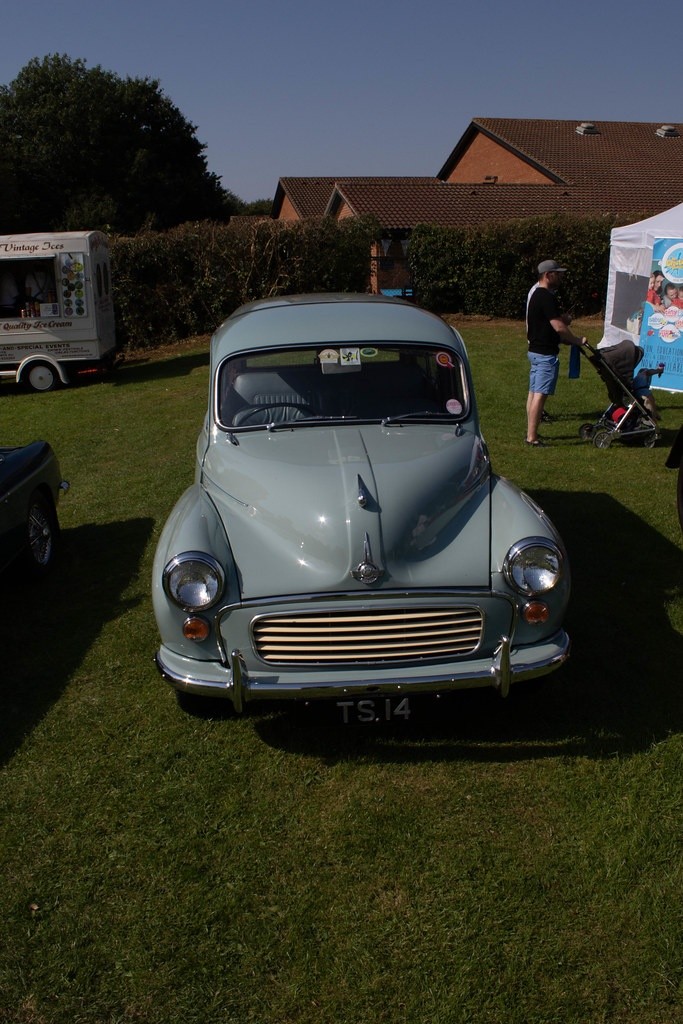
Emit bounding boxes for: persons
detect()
[634,362,665,421]
[527,280,572,422]
[0,265,19,319]
[525,259,569,445]
[25,258,52,304]
[647,270,683,308]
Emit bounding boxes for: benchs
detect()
[225,363,426,417]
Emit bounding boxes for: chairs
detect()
[231,403,316,426]
[349,395,435,417]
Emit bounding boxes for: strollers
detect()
[577,340,663,449]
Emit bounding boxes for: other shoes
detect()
[523,433,546,447]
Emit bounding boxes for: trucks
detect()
[0,231,118,393]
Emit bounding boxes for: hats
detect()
[537,260,568,274]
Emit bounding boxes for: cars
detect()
[152,290,570,708]
[0,438,70,571]
